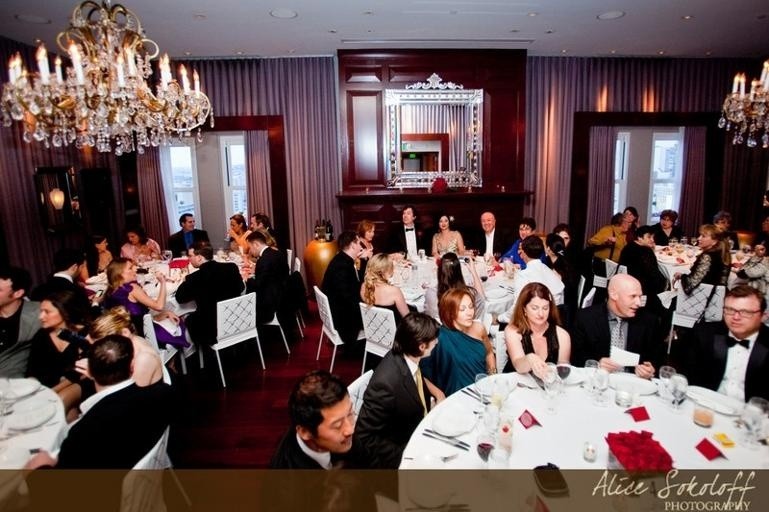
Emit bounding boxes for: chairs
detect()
[1,236,768,511]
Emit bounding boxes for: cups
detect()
[391,247,519,299]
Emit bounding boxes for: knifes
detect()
[420,428,471,453]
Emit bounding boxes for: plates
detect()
[430,402,477,438]
[402,469,457,506]
[1,378,55,470]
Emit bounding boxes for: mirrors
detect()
[378,73,487,189]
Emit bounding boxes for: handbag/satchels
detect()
[690,314,705,336]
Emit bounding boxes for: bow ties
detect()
[728,333,751,351]
[405,227,414,233]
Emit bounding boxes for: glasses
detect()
[721,303,764,320]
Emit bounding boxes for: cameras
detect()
[58,328,94,384]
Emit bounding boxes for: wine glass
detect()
[460,359,769,511]
[668,235,750,267]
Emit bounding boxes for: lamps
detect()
[0,1,217,159]
[31,165,79,233]
[718,61,768,152]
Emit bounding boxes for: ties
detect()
[413,368,429,418]
[610,315,625,366]
